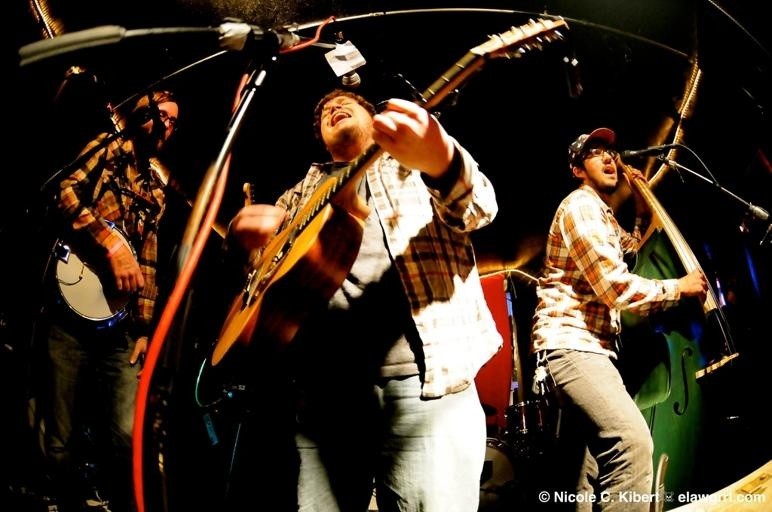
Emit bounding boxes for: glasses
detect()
[159,110,179,130]
[585,147,616,157]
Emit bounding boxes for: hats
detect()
[568,127,616,170]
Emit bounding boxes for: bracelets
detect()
[104,240,126,259]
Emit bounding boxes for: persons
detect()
[39,91,182,512]
[222,89,504,512]
[528,128,709,512]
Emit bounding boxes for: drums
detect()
[478,400,562,512]
[53,220,138,323]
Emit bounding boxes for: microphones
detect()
[335,32,362,89]
[620,143,677,166]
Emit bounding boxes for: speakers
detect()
[163,330,302,511]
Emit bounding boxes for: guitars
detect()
[211,15,570,366]
